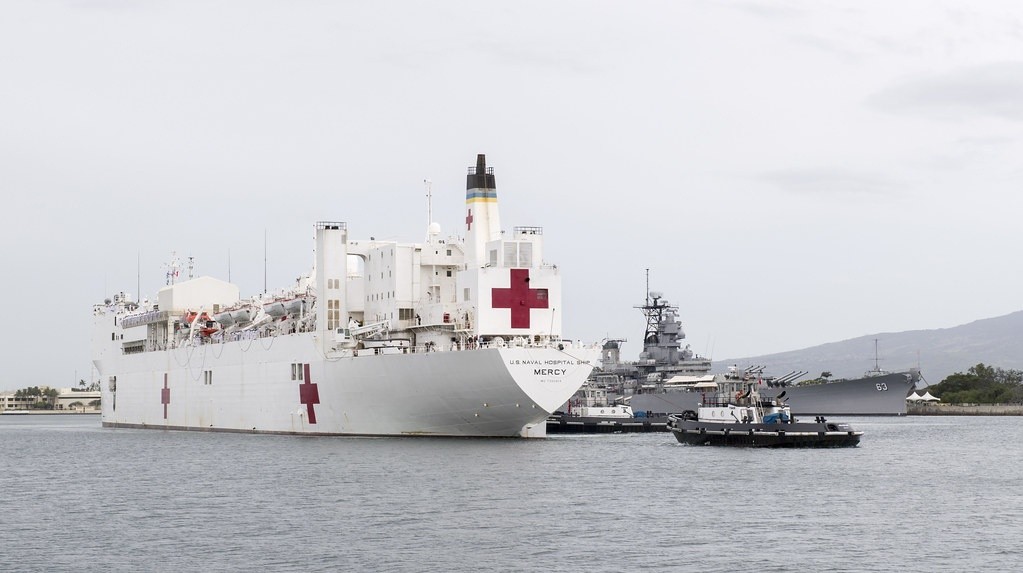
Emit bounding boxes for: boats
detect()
[546,315,922,427]
[94,154,595,438]
[666,382,865,448]
